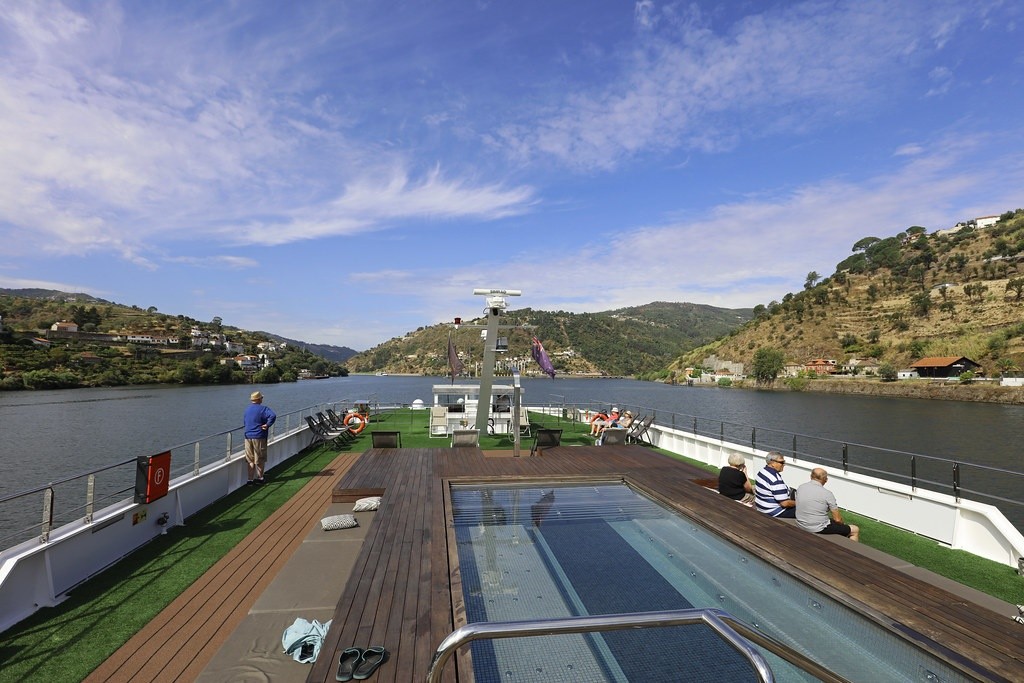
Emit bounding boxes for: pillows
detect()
[321,514,359,530]
[352,496,381,511]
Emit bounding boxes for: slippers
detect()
[335,646,364,681]
[353,646,385,679]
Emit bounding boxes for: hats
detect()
[249,391,264,401]
[612,407,618,412]
[626,411,631,415]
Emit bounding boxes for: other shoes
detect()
[258,476,266,483]
[247,481,254,486]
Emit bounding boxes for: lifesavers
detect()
[344,413,366,435]
[590,414,608,433]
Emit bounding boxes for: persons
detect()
[794,468,859,542]
[590,407,632,440]
[242,391,276,486]
[753,451,798,518]
[718,453,756,510]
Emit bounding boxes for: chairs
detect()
[315,411,353,441]
[601,409,641,430]
[596,428,630,446]
[303,415,347,450]
[430,407,449,438]
[371,431,402,448]
[450,430,479,447]
[627,415,656,445]
[529,429,563,455]
[325,409,356,441]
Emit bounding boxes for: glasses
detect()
[772,460,785,465]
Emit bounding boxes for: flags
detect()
[531,335,556,381]
[447,335,465,379]
